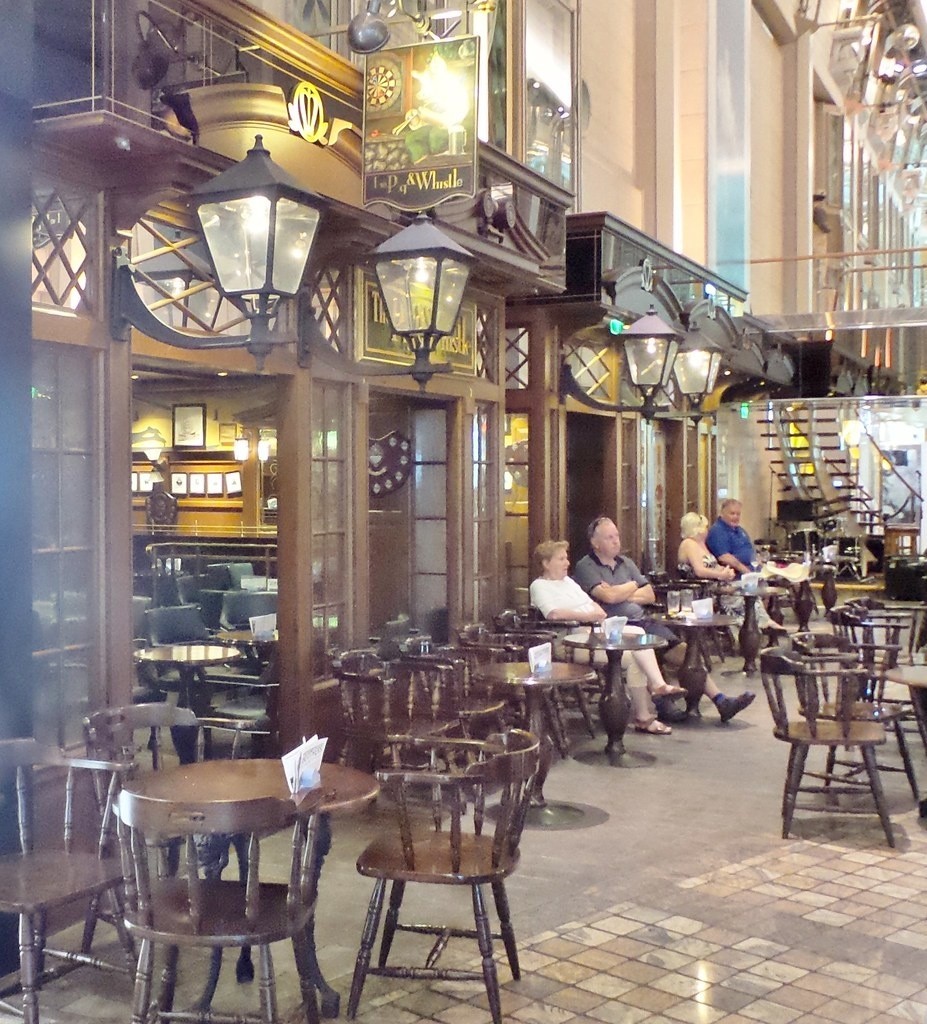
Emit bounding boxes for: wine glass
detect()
[759,550,770,567]
[750,553,760,572]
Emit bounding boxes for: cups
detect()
[667,590,681,615]
[680,589,693,612]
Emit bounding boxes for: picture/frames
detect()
[131,470,243,496]
[171,402,207,449]
[219,422,238,444]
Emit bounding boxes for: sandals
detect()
[651,684,688,701]
[635,717,673,735]
[660,709,687,722]
[718,691,756,722]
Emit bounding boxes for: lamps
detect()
[143,431,170,482]
[296,208,477,397]
[619,319,725,427]
[885,22,926,99]
[840,415,869,449]
[235,428,253,465]
[111,134,332,372]
[560,303,688,424]
[131,9,184,91]
[347,0,432,56]
[259,438,270,465]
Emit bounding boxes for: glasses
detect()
[591,516,610,537]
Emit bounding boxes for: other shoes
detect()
[758,621,786,635]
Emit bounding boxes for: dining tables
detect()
[885,666,927,819]
[559,633,669,769]
[764,573,817,634]
[716,586,790,684]
[213,629,281,711]
[118,758,381,1024]
[648,610,750,733]
[134,646,240,767]
[468,662,598,760]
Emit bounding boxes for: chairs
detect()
[1,737,140,1024]
[845,597,927,728]
[332,537,839,812]
[133,562,281,753]
[118,788,326,1024]
[81,703,259,958]
[31,590,88,706]
[760,646,896,850]
[348,729,541,1024]
[829,606,927,753]
[791,632,921,804]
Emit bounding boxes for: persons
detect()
[572,516,756,724]
[705,499,791,635]
[677,511,787,656]
[526,539,689,733]
